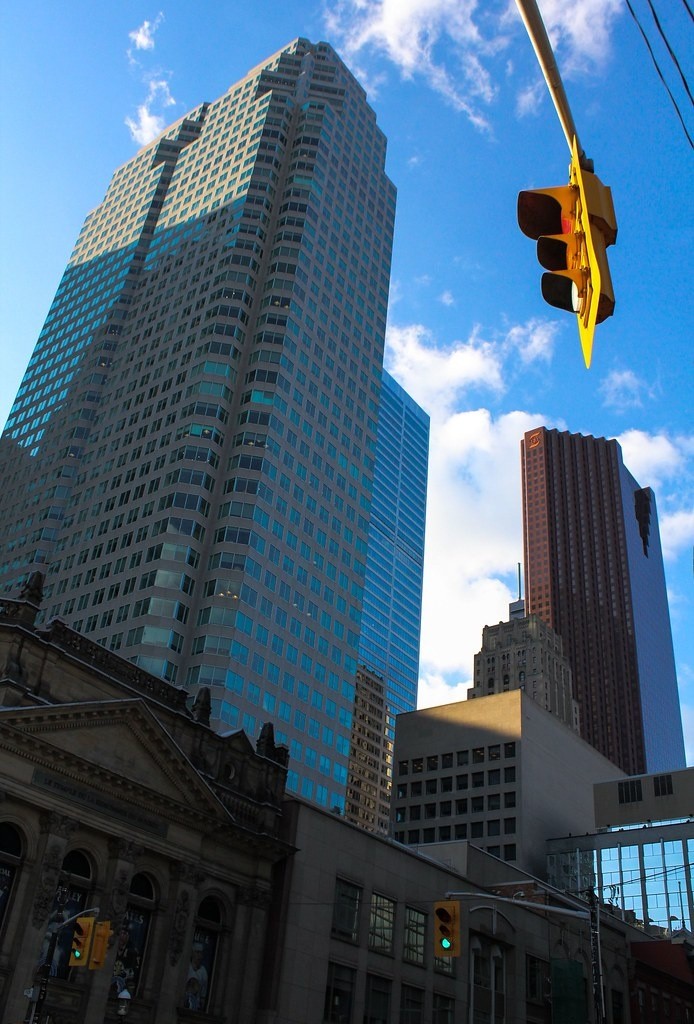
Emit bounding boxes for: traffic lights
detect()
[69,916,93,967]
[434,901,460,958]
[516,169,619,325]
